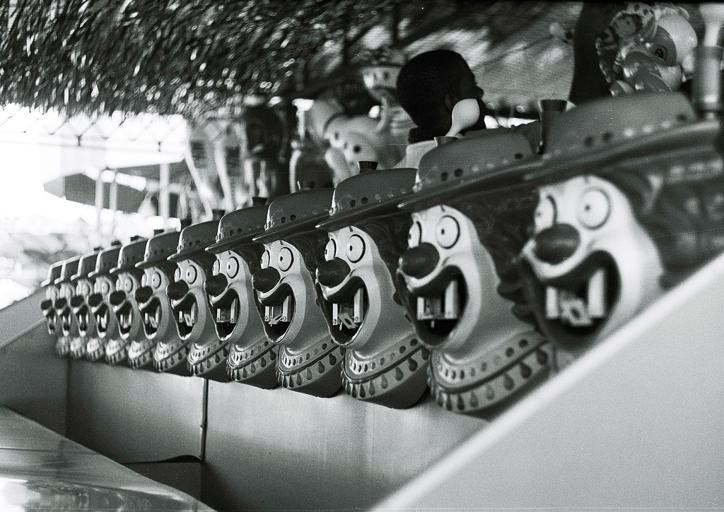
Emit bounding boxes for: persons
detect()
[395,50,488,142]
[40,90,724,420]
